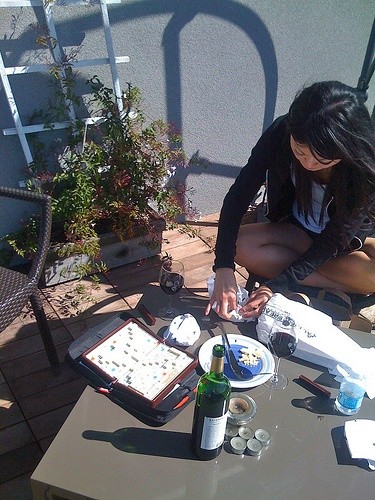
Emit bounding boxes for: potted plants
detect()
[0,0,218,315]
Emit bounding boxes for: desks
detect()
[31,285,375,500]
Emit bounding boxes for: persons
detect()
[206,80,375,319]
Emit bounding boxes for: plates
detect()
[199,334,275,388]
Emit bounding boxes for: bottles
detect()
[191,344,230,460]
[335,368,366,415]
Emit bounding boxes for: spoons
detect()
[217,322,253,381]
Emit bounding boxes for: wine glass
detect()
[264,316,298,390]
[158,260,185,321]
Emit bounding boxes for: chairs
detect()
[246,167,375,331]
[0,185,62,370]
